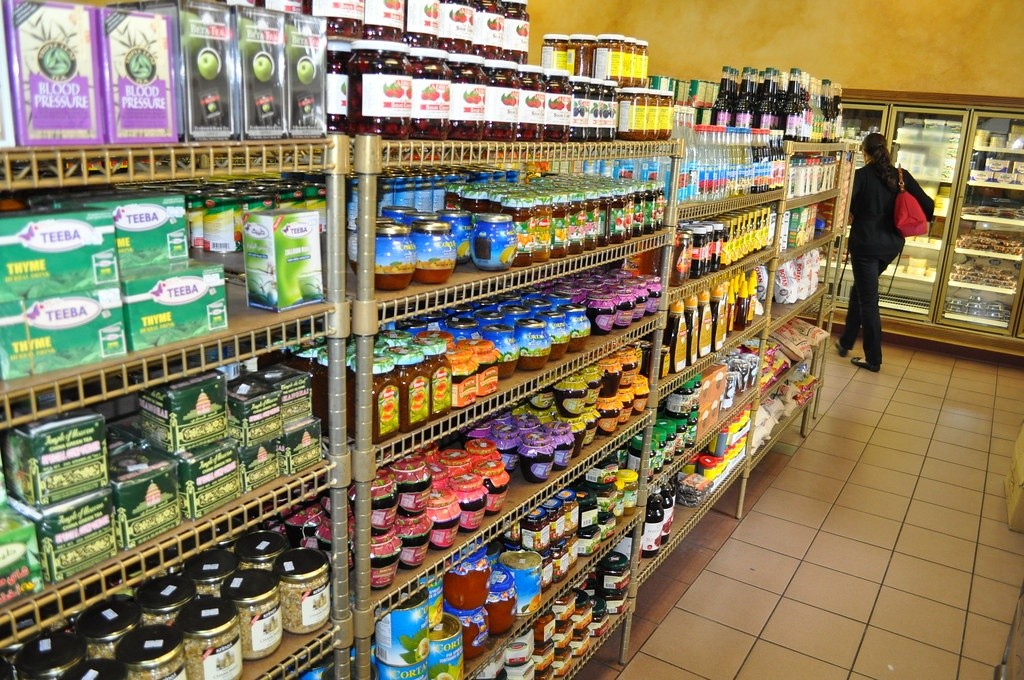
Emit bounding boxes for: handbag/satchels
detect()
[895,190,928,237]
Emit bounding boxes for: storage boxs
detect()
[0,0,328,148]
[1004,421,1024,533]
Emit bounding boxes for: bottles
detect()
[613,65,843,566]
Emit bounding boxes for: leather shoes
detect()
[851,356,871,370]
[835,339,848,356]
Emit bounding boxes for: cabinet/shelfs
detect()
[0,129,855,680]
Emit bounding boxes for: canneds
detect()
[0,343,702,680]
[269,273,662,445]
[607,221,724,286]
[214,0,674,141]
[648,76,720,125]
[172,167,665,290]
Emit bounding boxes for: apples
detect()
[199,53,313,84]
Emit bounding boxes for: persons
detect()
[834,132,934,372]
